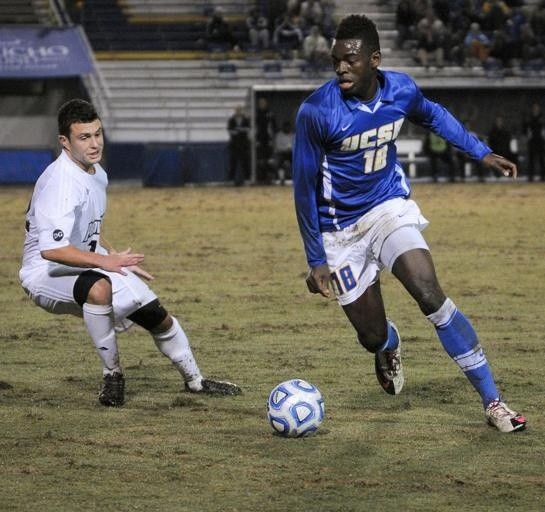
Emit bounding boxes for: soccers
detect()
[267,379,325,438]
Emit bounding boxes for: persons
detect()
[375,1,545,78]
[228,94,295,186]
[423,101,545,183]
[19,100,241,405]
[202,2,338,76]
[291,15,528,433]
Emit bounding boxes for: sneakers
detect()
[374,322,403,394]
[98,366,124,407]
[486,401,526,432]
[185,377,242,397]
